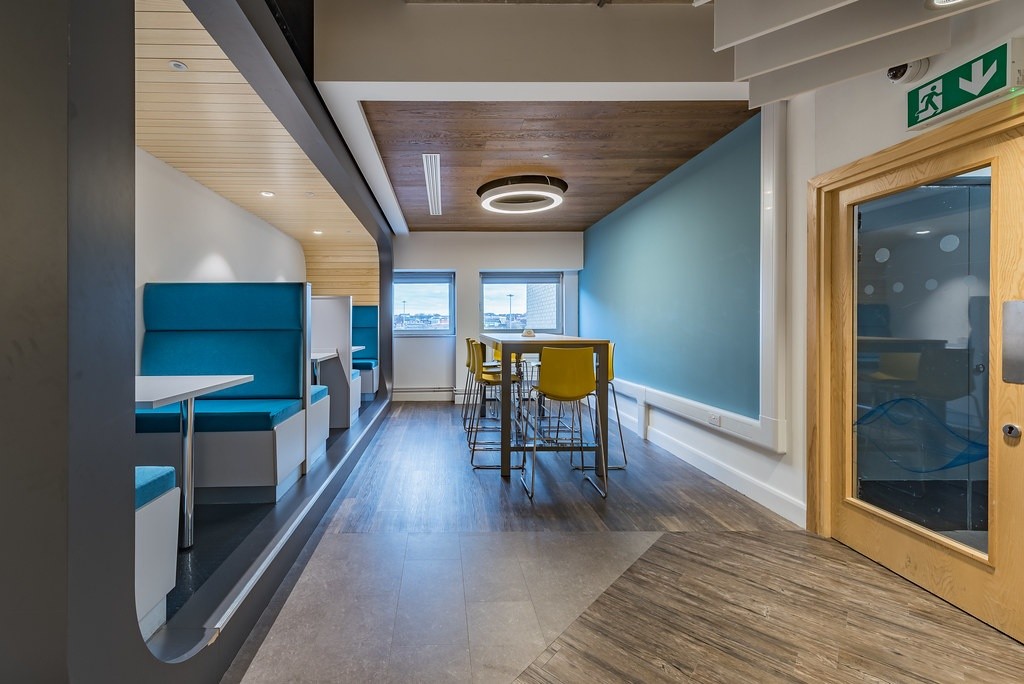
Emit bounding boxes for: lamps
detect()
[478,176,566,215]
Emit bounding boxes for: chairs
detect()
[352,305,382,401]
[135,466,180,644]
[311,385,332,463]
[140,281,307,505]
[460,336,628,472]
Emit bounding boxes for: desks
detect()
[310,351,339,384]
[857,331,976,452]
[135,375,255,550]
[478,331,610,479]
